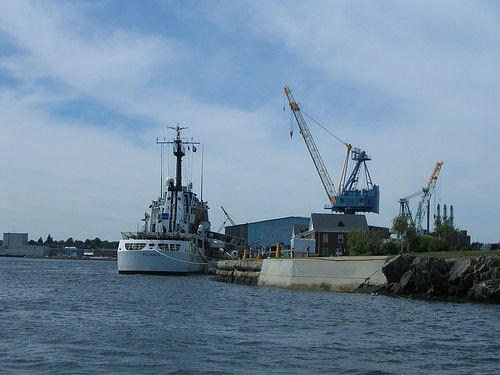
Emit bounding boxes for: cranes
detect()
[398,160,445,238]
[282,86,380,216]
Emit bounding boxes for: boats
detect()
[117,122,245,274]
